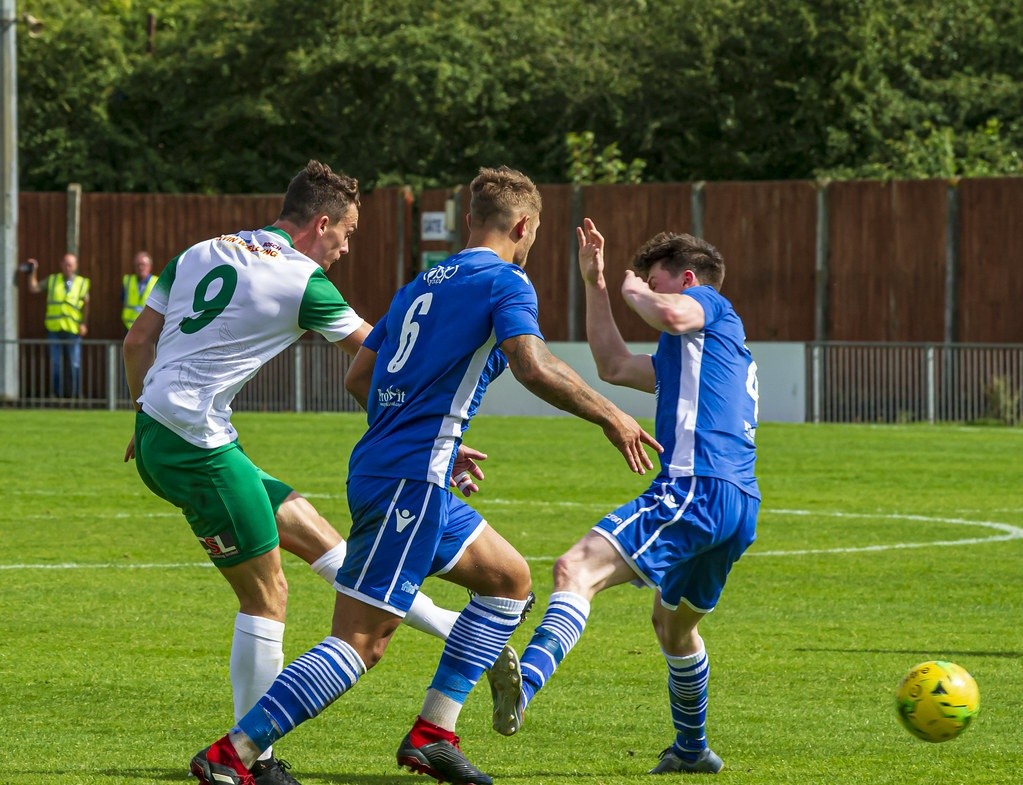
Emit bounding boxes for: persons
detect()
[120,250,160,408]
[26,254,91,406]
[123,160,532,785]
[190,166,663,785]
[484,216,761,775]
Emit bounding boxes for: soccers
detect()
[895,660,982,744]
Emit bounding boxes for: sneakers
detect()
[647,744,723,773]
[463,588,536,629]
[189,743,256,785]
[396,728,494,785]
[487,644,526,735]
[248,749,302,785]
